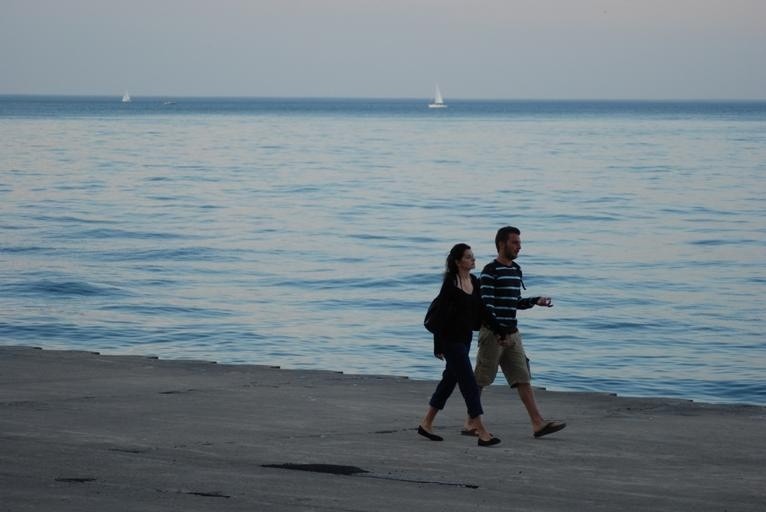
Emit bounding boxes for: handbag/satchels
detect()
[424,296,437,331]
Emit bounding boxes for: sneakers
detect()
[417,425,443,441]
[477,438,501,447]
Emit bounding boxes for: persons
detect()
[459,224,568,439]
[416,242,506,447]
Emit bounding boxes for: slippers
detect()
[533,421,567,437]
[461,424,493,437]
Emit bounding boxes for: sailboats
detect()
[121,88,131,104]
[427,83,448,109]
